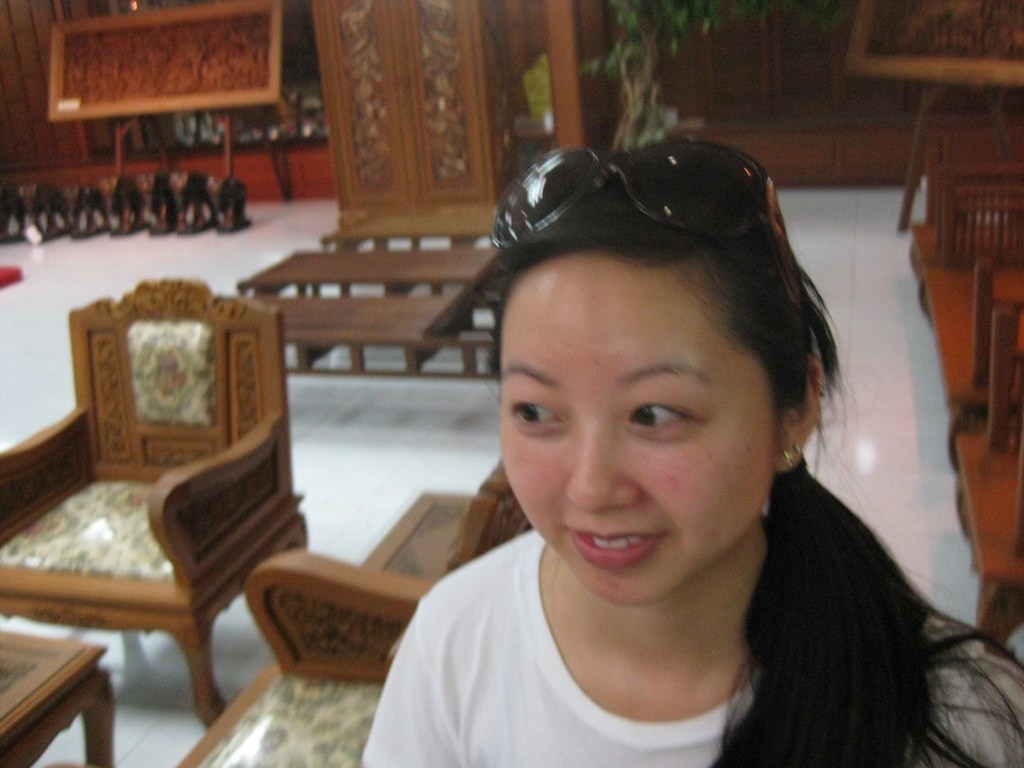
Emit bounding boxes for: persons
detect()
[362,138,1024,768]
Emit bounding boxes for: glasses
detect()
[490,138,784,244]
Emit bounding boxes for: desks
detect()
[358,488,479,582]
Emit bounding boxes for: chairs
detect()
[899,143,1023,647]
[0,280,312,725]
[183,465,512,766]
[1,632,118,768]
